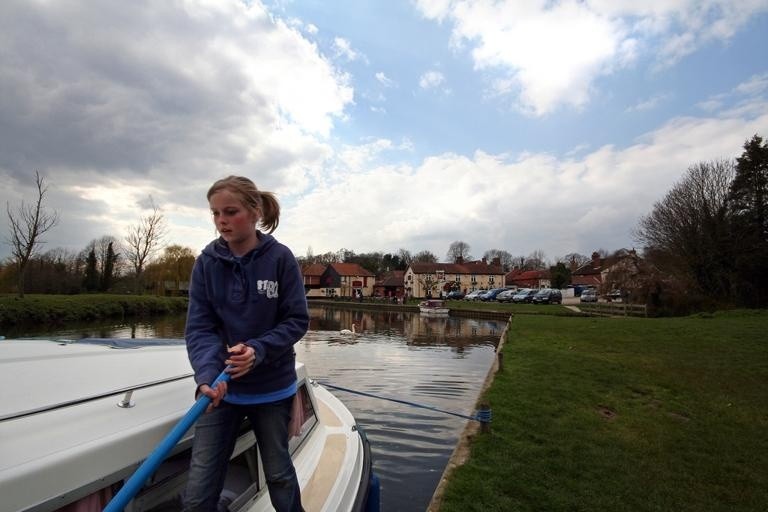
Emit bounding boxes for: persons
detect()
[184,174,309,511]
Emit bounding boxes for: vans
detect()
[480,288,507,302]
[512,288,539,303]
[532,288,562,304]
[580,289,598,302]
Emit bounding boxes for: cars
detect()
[446,291,465,300]
[603,289,621,303]
[496,289,517,303]
[463,289,488,302]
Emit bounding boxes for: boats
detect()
[0,332,382,511]
[419,299,450,314]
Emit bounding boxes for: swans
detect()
[340,324,357,335]
